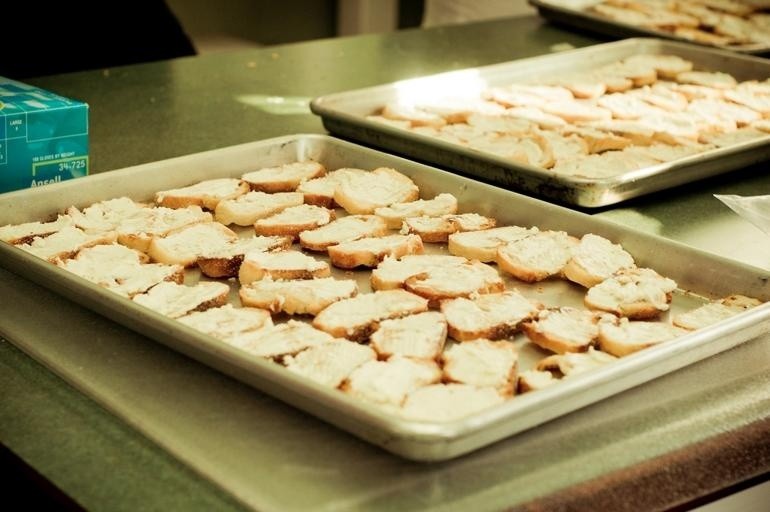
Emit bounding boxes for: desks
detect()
[0,15,769,512]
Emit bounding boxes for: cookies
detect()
[552,0,770,52]
[374,55,769,178]
[0,161,765,425]
